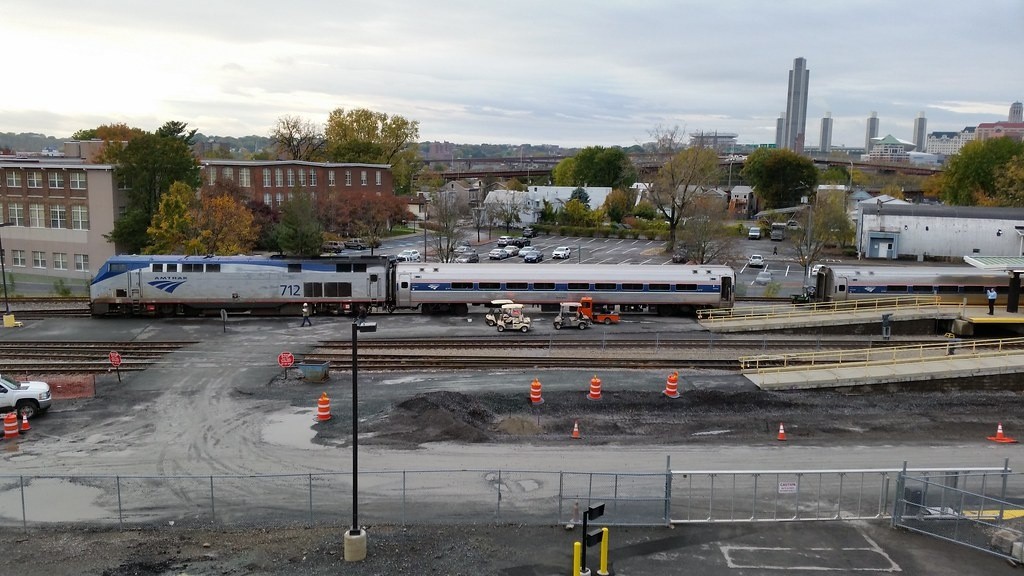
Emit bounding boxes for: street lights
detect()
[344,321,378,563]
[0,222,16,328]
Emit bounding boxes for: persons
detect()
[986,288,997,315]
[299,303,312,327]
[773,246,778,255]
[357,304,367,325]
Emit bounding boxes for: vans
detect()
[746,220,801,241]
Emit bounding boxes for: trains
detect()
[814,265,1024,308]
[88,254,737,320]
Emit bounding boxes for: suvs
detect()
[397,249,421,262]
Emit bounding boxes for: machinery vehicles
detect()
[577,295,619,324]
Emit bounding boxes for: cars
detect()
[811,265,824,276]
[453,227,571,264]
[322,236,379,253]
[756,272,773,285]
[748,254,764,267]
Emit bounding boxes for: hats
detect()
[302,303,308,306]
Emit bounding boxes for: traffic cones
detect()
[21,412,32,431]
[0,374,52,420]
[995,423,1007,441]
[572,421,582,439]
[777,423,787,441]
